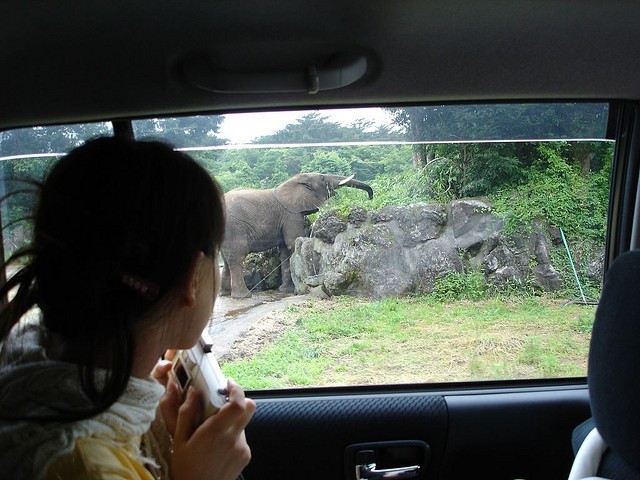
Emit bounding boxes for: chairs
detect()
[567,249,639,480]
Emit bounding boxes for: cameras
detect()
[164,329,229,425]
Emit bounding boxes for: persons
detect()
[0,135,256,480]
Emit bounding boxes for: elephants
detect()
[218,172,373,298]
